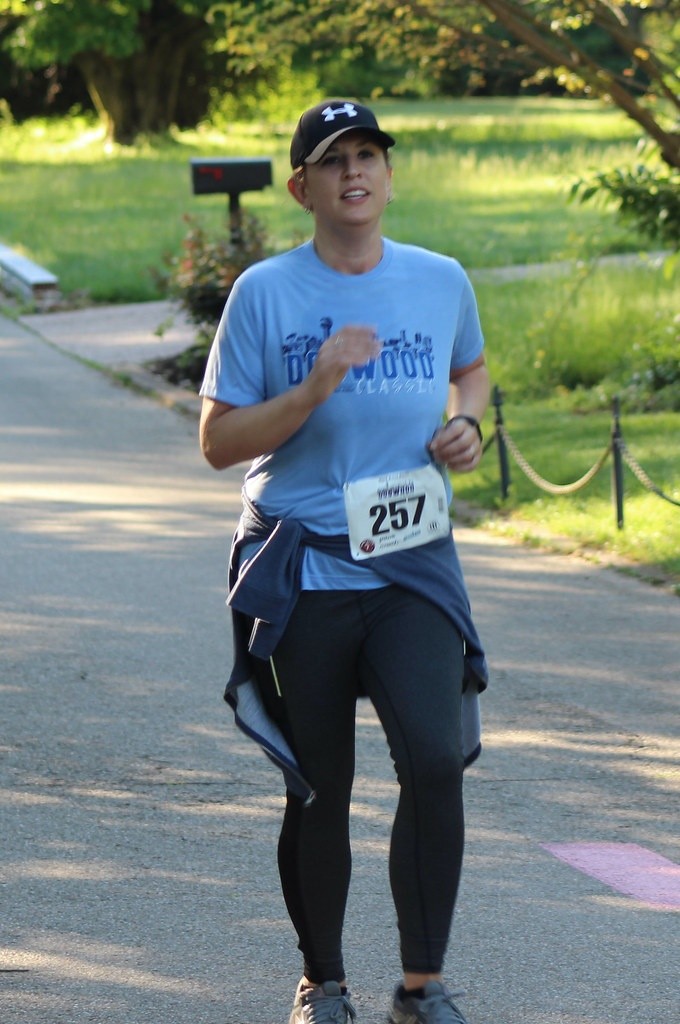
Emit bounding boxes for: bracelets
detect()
[447,415,483,442]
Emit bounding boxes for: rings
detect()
[334,336,343,346]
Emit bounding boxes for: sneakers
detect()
[391,980,466,1024]
[289,979,357,1024]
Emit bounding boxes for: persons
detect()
[197,101,491,1024]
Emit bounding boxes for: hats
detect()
[290,101,395,170]
